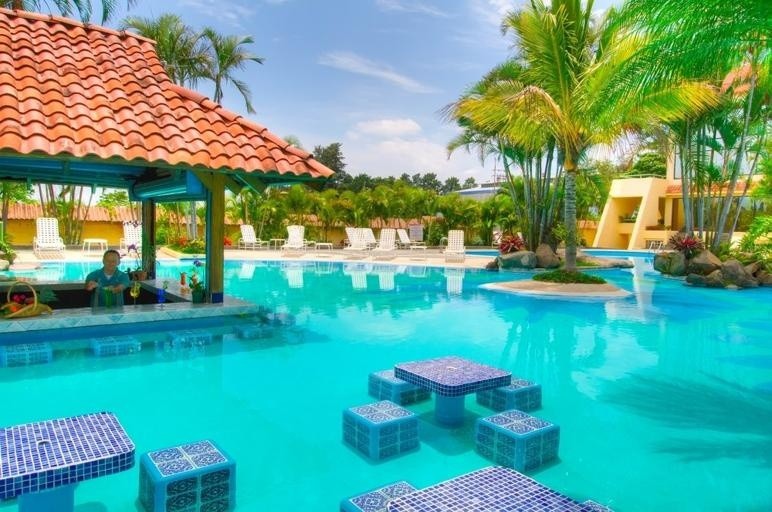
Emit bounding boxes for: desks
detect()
[270,239,284,251]
[316,242,333,252]
[385,464,594,511]
[393,356,511,425]
[82,239,109,253]
[0,412,135,512]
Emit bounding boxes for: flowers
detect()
[188,261,205,293]
[126,244,147,271]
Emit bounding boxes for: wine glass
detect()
[180,272,186,289]
[157,288,166,311]
[130,282,142,309]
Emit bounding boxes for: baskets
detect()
[0,281,53,319]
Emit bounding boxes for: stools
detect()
[341,479,418,512]
[139,437,235,512]
[343,369,559,474]
[583,498,616,512]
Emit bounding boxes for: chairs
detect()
[33,217,67,255]
[443,229,466,263]
[281,225,315,254]
[491,222,504,248]
[343,227,428,260]
[238,224,267,251]
[120,221,141,255]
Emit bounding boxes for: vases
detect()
[193,291,205,303]
[131,271,147,280]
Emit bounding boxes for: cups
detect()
[163,281,169,289]
